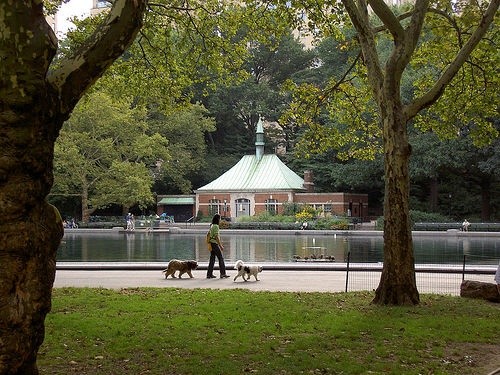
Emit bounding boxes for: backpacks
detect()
[206,231,216,252]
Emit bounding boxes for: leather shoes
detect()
[220,274,231,278]
[206,274,217,279]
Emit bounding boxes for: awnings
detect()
[158,198,195,206]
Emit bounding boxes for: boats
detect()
[295,245,335,262]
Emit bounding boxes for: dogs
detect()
[163,259,198,279]
[234,260,262,282]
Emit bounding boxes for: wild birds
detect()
[293,254,335,260]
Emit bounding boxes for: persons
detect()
[126,213,135,230]
[207,213,230,278]
[63,218,78,229]
[494,262,500,294]
[462,219,470,232]
[140,213,175,226]
[301,220,308,230]
